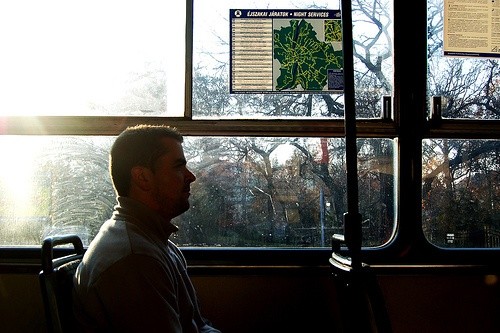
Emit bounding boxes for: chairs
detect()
[38,235,84,333]
[332,234,393,333]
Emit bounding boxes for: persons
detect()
[71,124,222,333]
